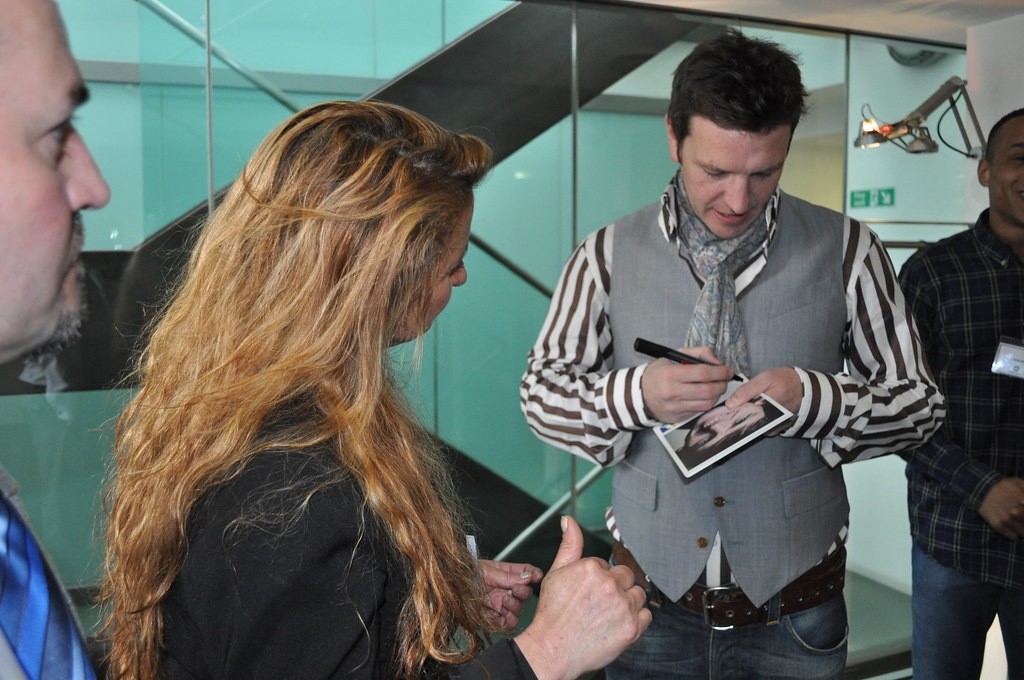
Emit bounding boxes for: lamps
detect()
[853,75,986,159]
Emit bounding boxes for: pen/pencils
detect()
[632,336,745,383]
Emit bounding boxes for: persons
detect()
[677,397,773,468]
[521,39,945,678]
[901,109,1024,679]
[98,103,652,679]
[0,1,112,678]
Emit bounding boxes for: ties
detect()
[0,488,94,680]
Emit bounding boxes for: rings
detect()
[508,589,512,594]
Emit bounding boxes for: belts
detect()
[612,539,846,632]
